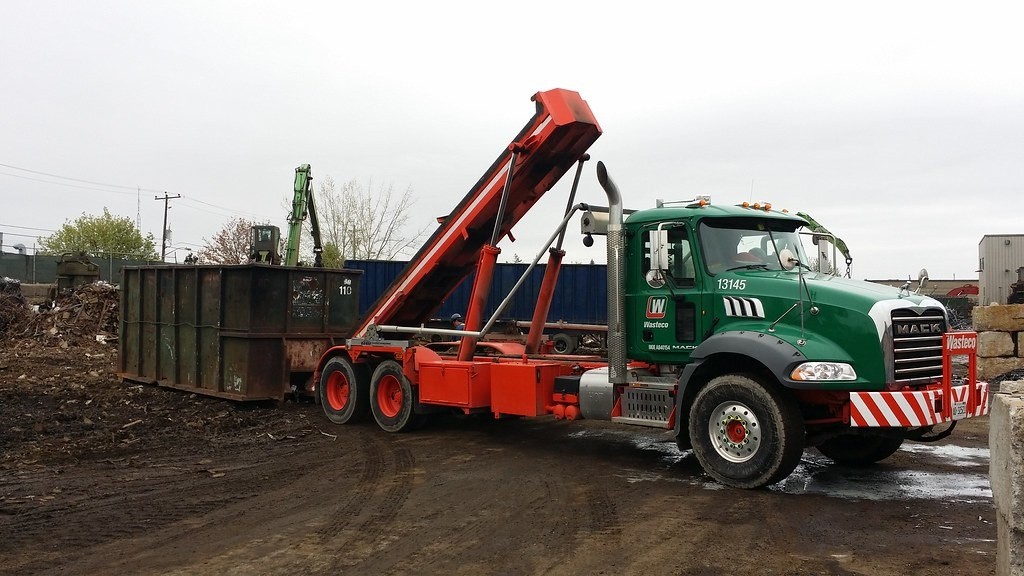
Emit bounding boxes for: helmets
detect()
[450,313,461,322]
[749,247,766,259]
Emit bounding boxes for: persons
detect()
[450,314,465,351]
[728,248,770,264]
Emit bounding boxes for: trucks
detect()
[308,89,989,492]
